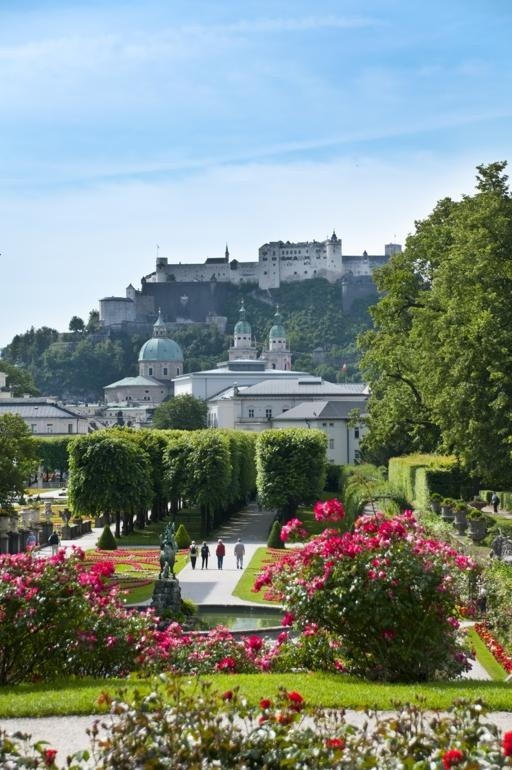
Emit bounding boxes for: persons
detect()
[215,539,225,570]
[48,531,61,555]
[233,538,246,569]
[31,471,68,483]
[201,541,211,570]
[491,491,500,513]
[27,532,37,547]
[189,540,200,570]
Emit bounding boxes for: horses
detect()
[157,535,178,580]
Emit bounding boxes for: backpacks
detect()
[191,545,196,554]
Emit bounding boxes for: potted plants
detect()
[30,502,41,521]
[429,492,488,545]
